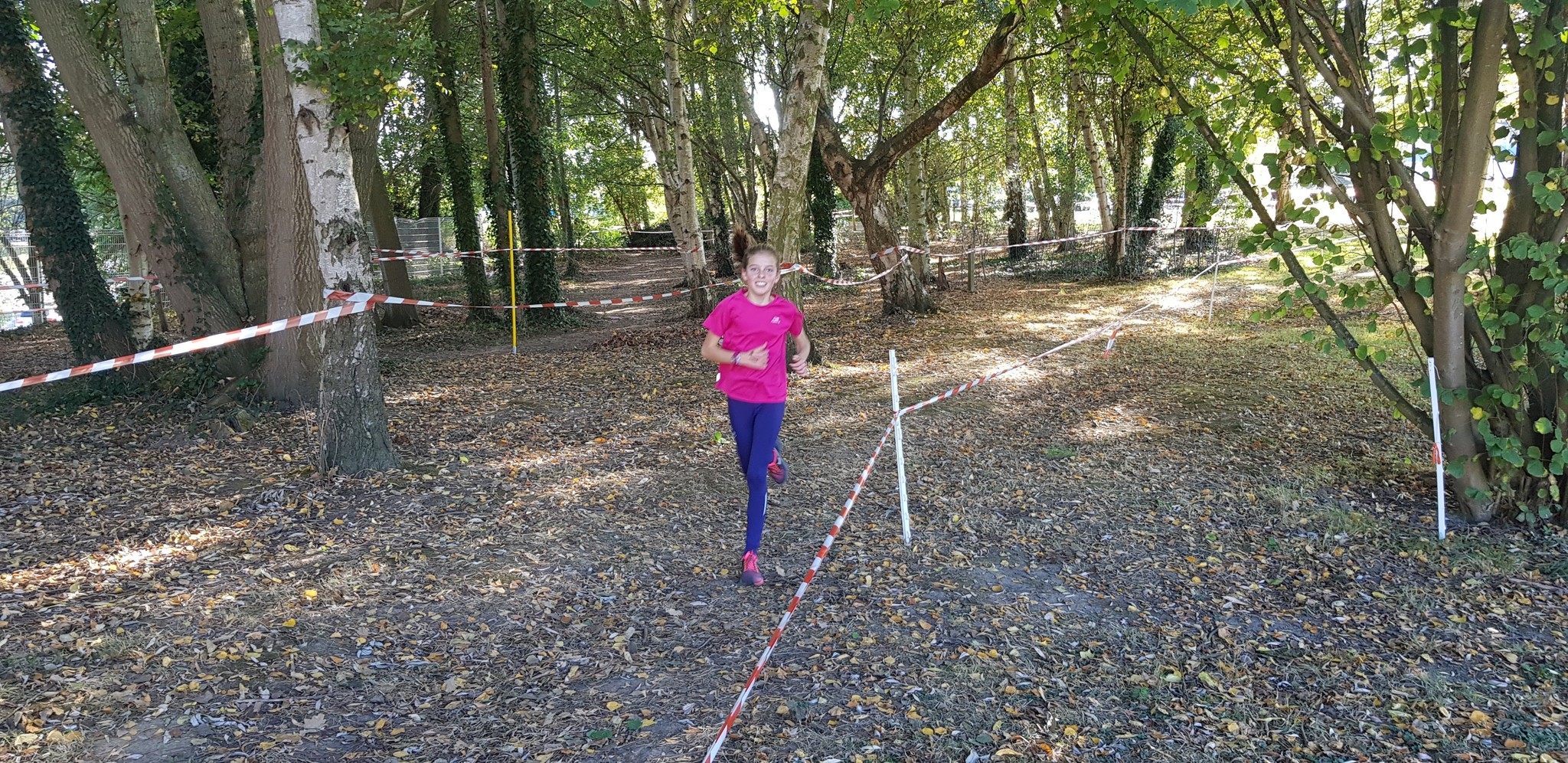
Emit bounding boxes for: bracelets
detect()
[731,351,740,366]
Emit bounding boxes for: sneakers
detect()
[741,551,763,586]
[767,436,788,484]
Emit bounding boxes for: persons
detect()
[699,225,812,586]
